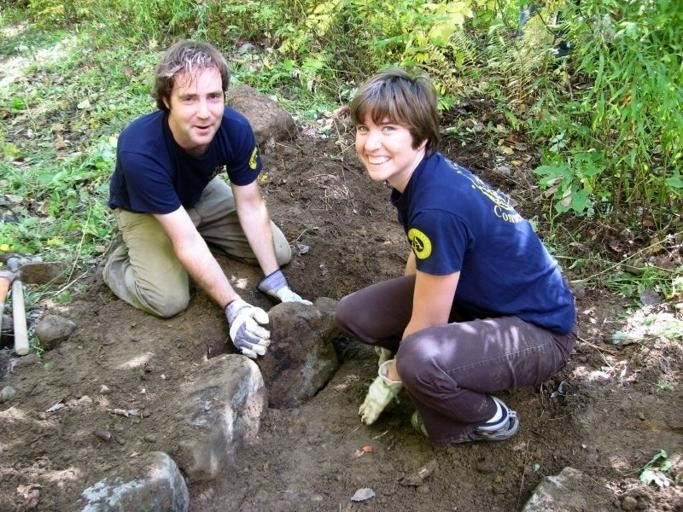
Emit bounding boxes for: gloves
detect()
[255,268,313,305]
[221,298,270,359]
[357,345,402,426]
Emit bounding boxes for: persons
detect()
[103,39,313,360]
[331,68,577,445]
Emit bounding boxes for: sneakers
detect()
[100,226,122,265]
[410,405,519,445]
